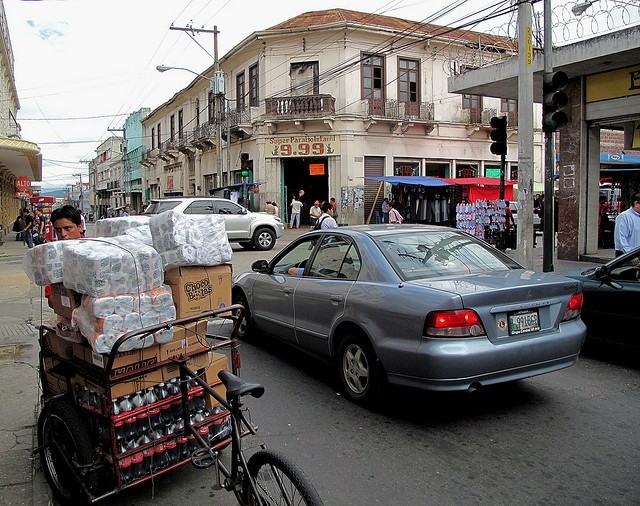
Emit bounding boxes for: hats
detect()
[314,200,320,204]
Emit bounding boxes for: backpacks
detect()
[312,217,328,247]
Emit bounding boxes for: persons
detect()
[13,216,24,241]
[43,205,91,309]
[131,207,136,216]
[36,208,46,244]
[139,205,147,215]
[613,193,640,266]
[382,198,389,223]
[23,209,34,249]
[330,198,338,220]
[315,202,339,229]
[265,201,275,215]
[505,199,512,249]
[291,194,296,202]
[121,207,130,217]
[534,192,545,249]
[386,202,404,224]
[271,202,279,217]
[289,267,305,278]
[78,209,86,236]
[260,261,267,268]
[289,193,303,229]
[309,200,322,223]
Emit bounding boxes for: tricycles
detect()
[485,222,543,252]
[38,304,323,506]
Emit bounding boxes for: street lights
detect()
[571,0,640,16]
[156,65,221,189]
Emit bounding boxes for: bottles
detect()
[120,395,136,435]
[117,437,133,486]
[150,429,170,468]
[164,421,180,464]
[143,387,161,428]
[174,417,188,460]
[194,409,209,448]
[207,406,231,436]
[108,399,123,437]
[75,385,104,446]
[139,431,153,473]
[168,379,183,421]
[157,381,173,422]
[196,378,206,410]
[132,391,146,435]
[187,379,195,415]
[187,416,196,453]
[128,432,144,477]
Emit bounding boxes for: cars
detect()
[231,224,588,404]
[143,198,284,250]
[509,202,542,225]
[550,245,640,369]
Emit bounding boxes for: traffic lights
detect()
[543,70,570,132]
[490,116,507,155]
[241,152,249,177]
